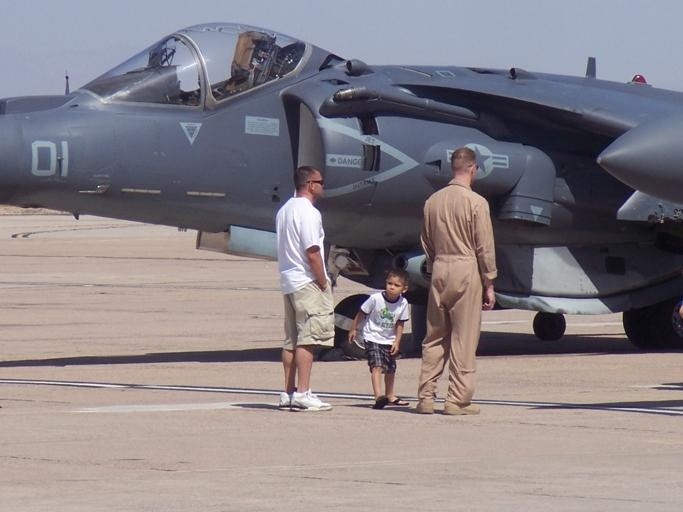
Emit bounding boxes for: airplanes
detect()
[1,21,683,360]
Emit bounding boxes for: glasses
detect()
[305,179,324,185]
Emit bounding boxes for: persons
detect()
[415,147,497,415]
[348,269,409,408]
[275,167,335,412]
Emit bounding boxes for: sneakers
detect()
[277,391,292,410]
[443,402,480,415]
[372,395,408,408]
[415,401,433,413]
[290,388,332,413]
